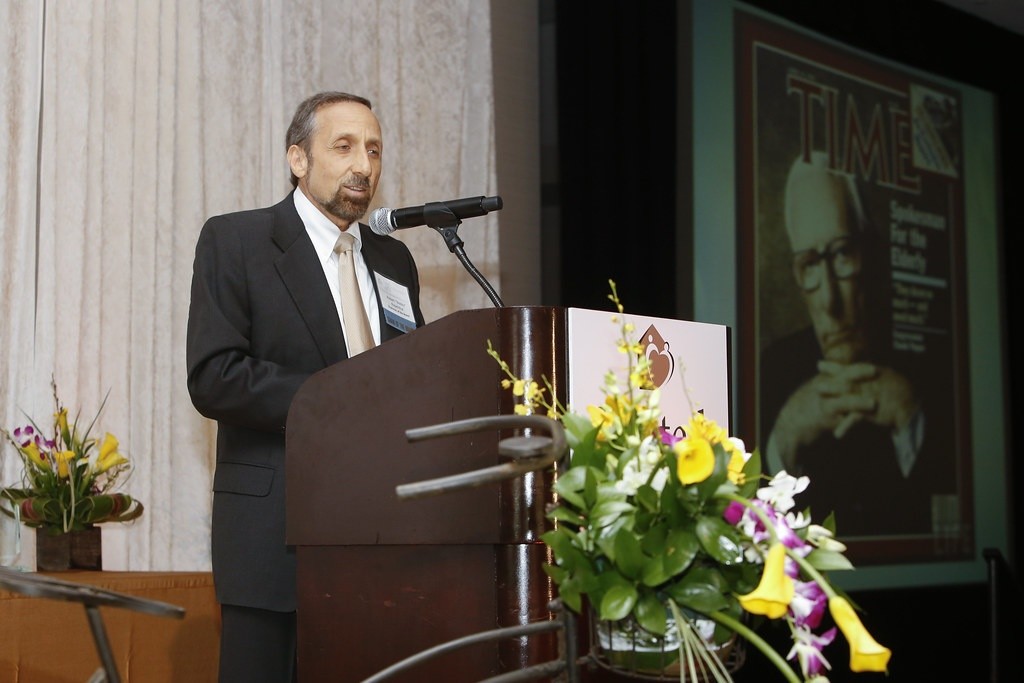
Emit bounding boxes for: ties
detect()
[333,232,377,359]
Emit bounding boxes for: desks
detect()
[0,570,221,683]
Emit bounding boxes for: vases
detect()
[594,605,741,677]
[37,526,102,572]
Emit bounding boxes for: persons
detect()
[760,153,954,536]
[187,92,426,683]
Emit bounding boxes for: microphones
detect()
[369,196,503,235]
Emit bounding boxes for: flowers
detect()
[0,371,144,536]
[486,279,891,683]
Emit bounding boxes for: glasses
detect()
[783,233,874,293]
